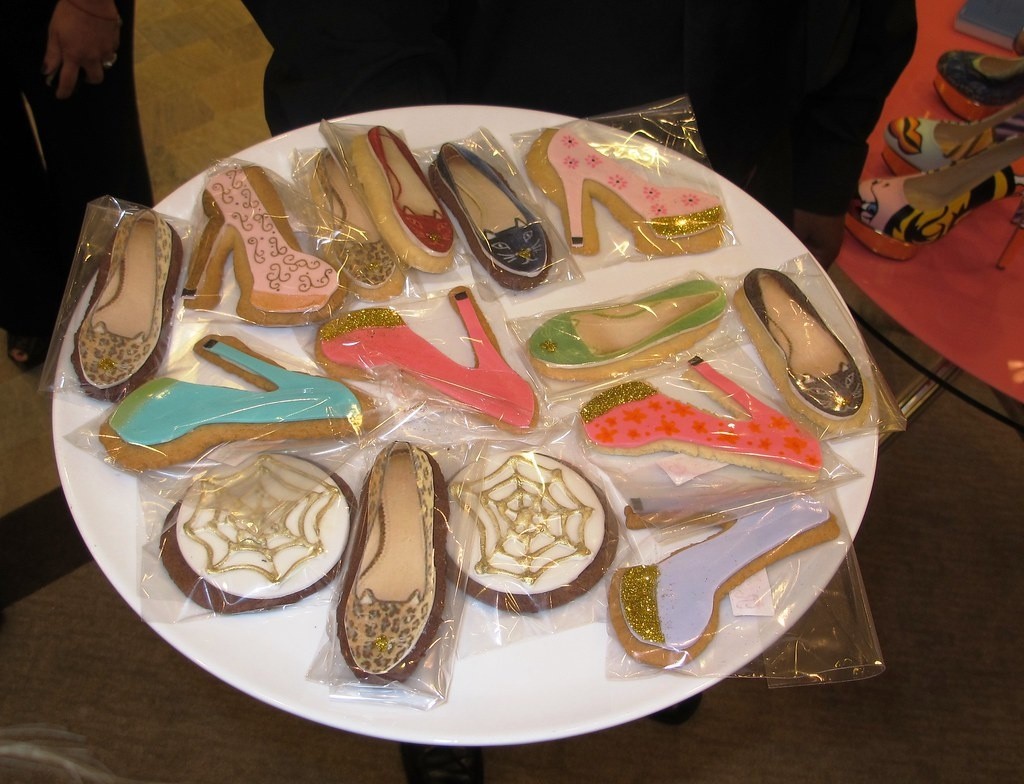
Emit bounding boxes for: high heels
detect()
[933,49,1024,122]
[883,97,1024,173]
[845,134,1024,270]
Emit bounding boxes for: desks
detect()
[827,0,1024,450]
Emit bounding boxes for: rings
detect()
[102,53,118,68]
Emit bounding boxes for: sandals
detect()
[7,333,51,373]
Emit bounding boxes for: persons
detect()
[246,0,917,784]
[0,0,153,371]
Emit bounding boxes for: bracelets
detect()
[71,3,119,27]
